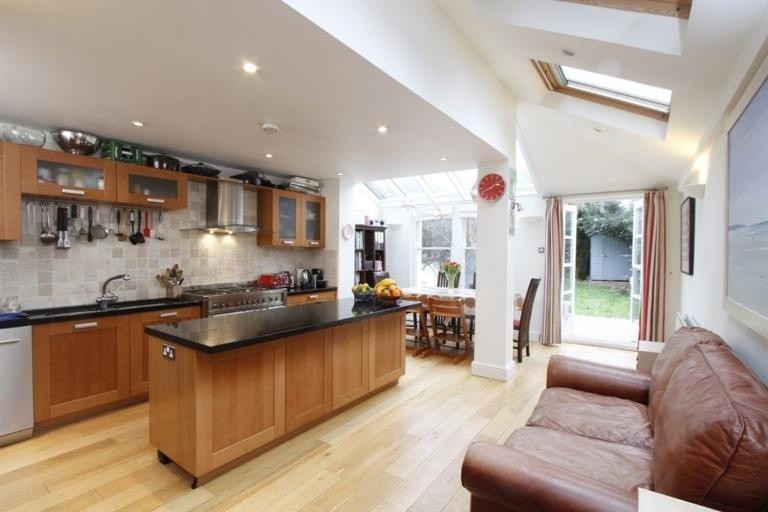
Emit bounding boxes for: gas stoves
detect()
[183,283,286,314]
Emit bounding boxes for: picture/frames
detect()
[680,196,695,275]
[721,54,768,341]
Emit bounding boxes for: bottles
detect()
[4,295,22,314]
[287,275,294,288]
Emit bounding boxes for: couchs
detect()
[461,325,768,512]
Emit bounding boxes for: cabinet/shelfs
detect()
[354,224,389,287]
[636,340,666,374]
[0,320,33,449]
[287,285,337,307]
[0,141,23,241]
[34,300,201,436]
[21,139,188,242]
[257,188,326,249]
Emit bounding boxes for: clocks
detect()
[478,173,506,202]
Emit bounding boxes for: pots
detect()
[141,150,182,171]
[181,161,221,178]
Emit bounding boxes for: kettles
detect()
[300,269,312,290]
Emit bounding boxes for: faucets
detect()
[102,274,132,297]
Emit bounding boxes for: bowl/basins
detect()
[352,292,370,302]
[1,124,46,147]
[376,293,400,302]
[50,128,101,158]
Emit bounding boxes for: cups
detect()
[295,268,302,288]
[38,166,51,183]
[69,172,83,187]
[131,182,150,196]
[54,167,69,186]
[96,178,104,191]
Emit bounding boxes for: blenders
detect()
[311,268,325,289]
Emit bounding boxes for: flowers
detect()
[442,259,460,274]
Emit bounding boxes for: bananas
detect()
[374,279,397,291]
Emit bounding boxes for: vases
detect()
[445,274,457,295]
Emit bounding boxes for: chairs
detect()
[373,271,542,365]
[437,271,461,288]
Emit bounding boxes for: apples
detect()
[353,283,372,292]
[375,285,395,296]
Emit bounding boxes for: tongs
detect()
[25,199,37,238]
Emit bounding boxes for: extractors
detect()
[177,181,261,235]
